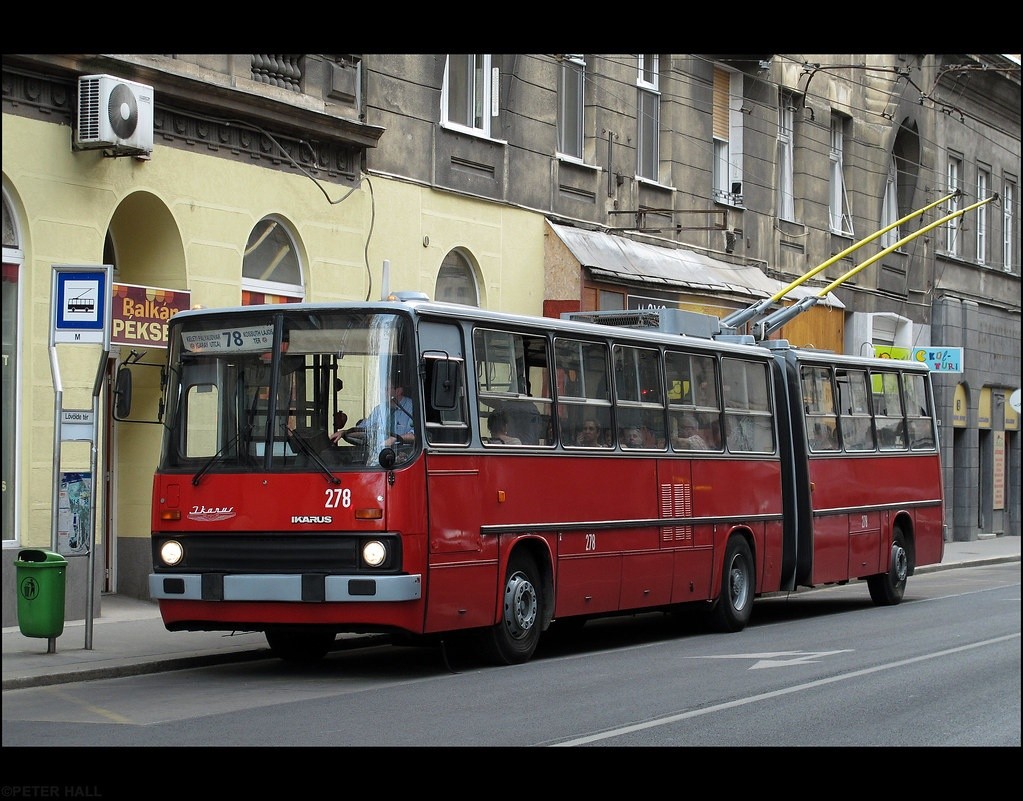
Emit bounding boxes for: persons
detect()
[329,374,416,448]
[488,375,754,452]
[812,419,935,449]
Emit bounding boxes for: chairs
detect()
[484,399,717,449]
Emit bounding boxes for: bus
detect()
[111,291,945,668]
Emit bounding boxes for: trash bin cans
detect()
[13,548,68,638]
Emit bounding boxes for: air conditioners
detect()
[69,73,153,158]
